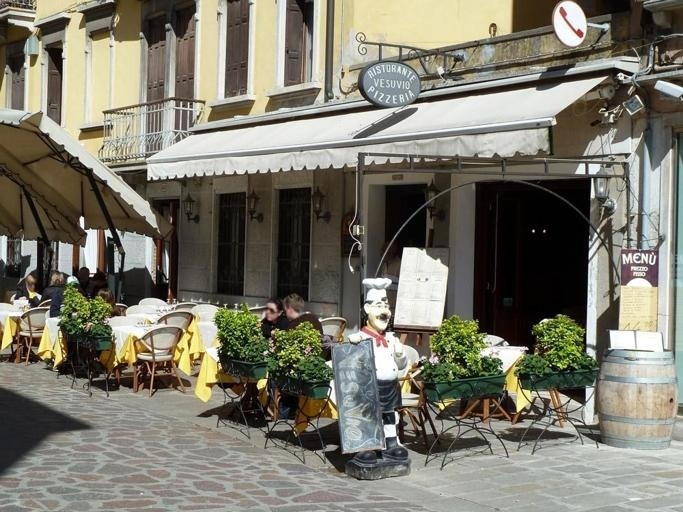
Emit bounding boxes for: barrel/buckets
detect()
[596,348,679,450]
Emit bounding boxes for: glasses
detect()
[265,306,276,315]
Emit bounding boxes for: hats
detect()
[67,275,80,285]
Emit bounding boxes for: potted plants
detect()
[515,315,600,391]
[57,281,91,343]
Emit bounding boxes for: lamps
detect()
[312,186,331,223]
[247,189,263,224]
[425,178,445,221]
[593,163,617,215]
[182,192,200,224]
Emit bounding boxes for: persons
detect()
[280,293,323,416]
[343,278,419,465]
[97,288,121,318]
[256,296,290,341]
[86,272,108,299]
[62,275,88,299]
[29,270,67,317]
[77,268,91,292]
[13,270,41,308]
